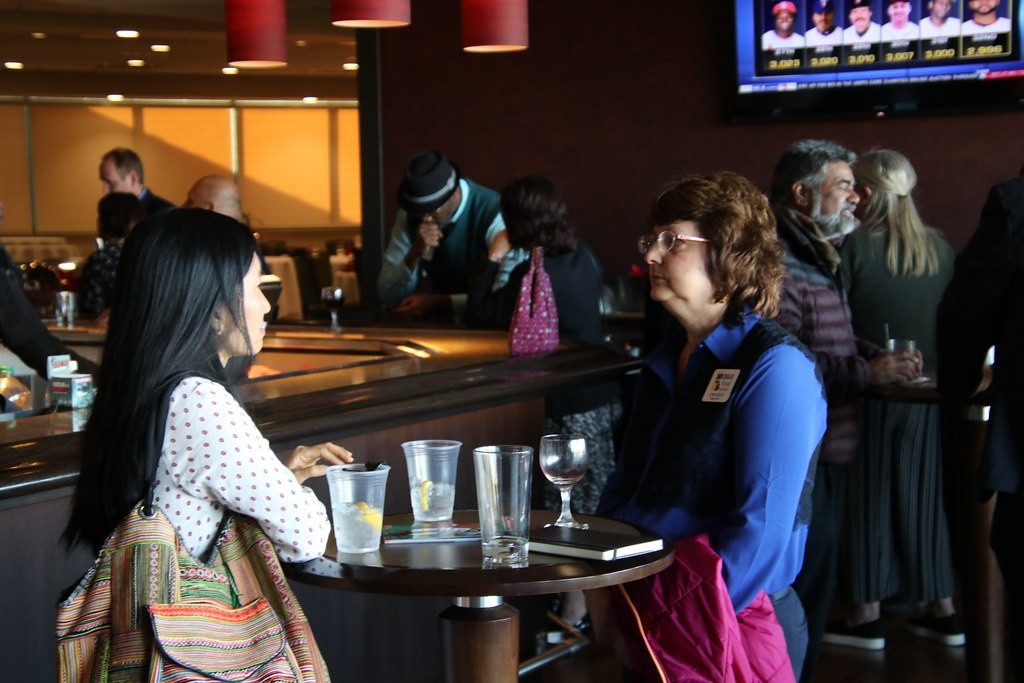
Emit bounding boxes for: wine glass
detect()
[539,433,592,531]
[320,287,344,332]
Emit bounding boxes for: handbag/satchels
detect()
[510,247,561,352]
[56,504,333,682]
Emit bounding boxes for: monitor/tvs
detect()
[714,0,1024,122]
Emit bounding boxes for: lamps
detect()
[224,0,288,68]
[461,0,528,53]
[330,0,411,27]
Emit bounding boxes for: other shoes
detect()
[905,605,968,647]
[822,619,886,647]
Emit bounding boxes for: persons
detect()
[378,151,510,332]
[490,177,621,640]
[762,0,1010,48]
[60,206,355,562]
[78,148,174,319]
[584,172,827,683]
[772,141,925,683]
[937,173,1023,683]
[184,173,242,220]
[822,150,966,649]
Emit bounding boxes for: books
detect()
[503,525,663,560]
[383,519,513,544]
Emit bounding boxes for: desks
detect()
[280,508,676,683]
[852,368,1007,683]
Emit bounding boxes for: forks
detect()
[341,461,387,473]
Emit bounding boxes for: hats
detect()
[393,150,458,211]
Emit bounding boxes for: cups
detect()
[326,463,392,555]
[885,339,916,356]
[401,439,463,523]
[472,444,534,564]
[55,291,75,325]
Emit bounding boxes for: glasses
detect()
[636,231,708,252]
